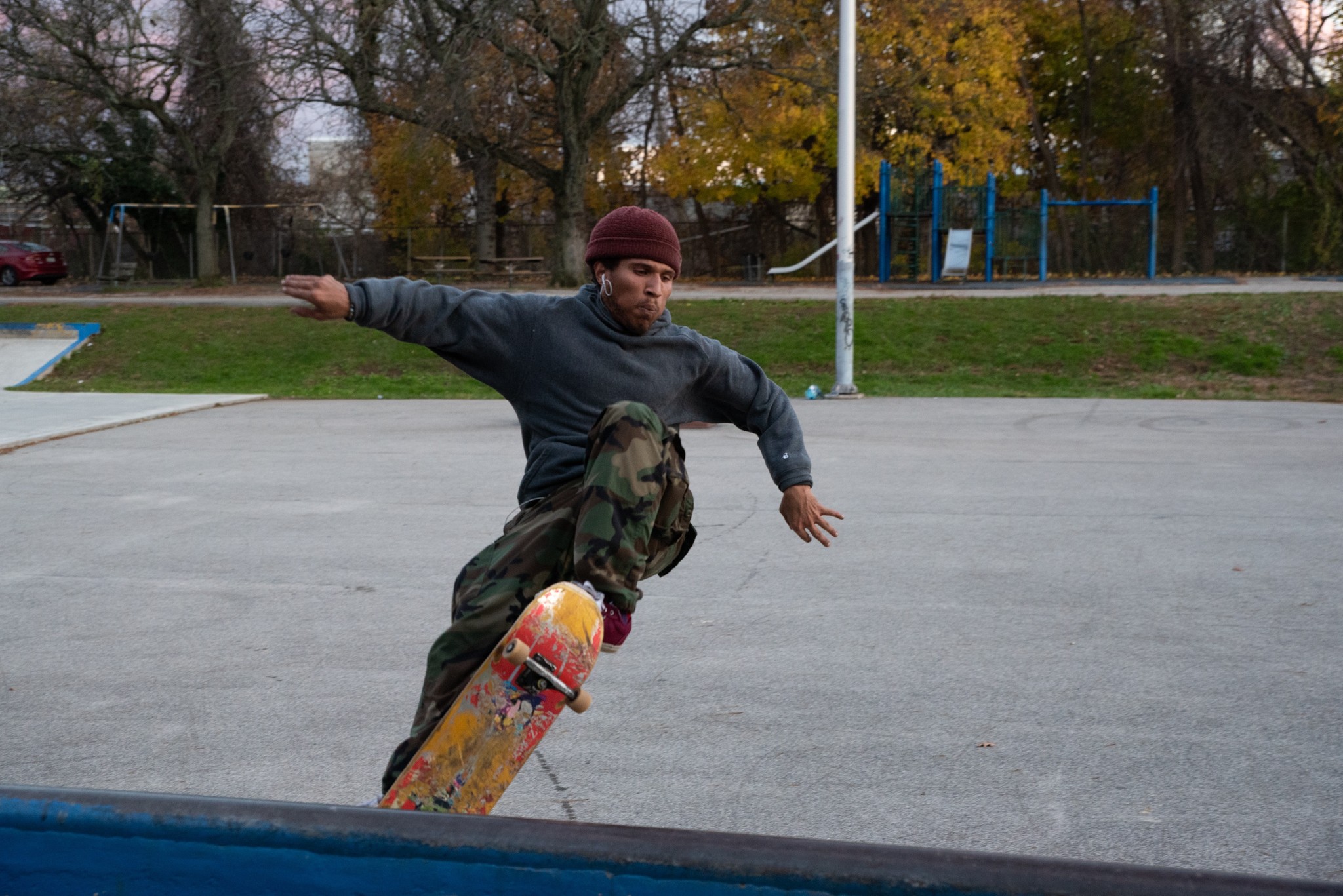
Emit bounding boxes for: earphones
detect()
[602,274,605,285]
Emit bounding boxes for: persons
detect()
[116,213,157,285]
[273,206,844,798]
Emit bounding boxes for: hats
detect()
[584,206,681,278]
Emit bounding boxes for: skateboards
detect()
[373,580,605,816]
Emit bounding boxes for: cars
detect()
[0,240,68,285]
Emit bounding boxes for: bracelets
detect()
[343,301,355,322]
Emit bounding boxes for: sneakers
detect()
[593,601,633,651]
